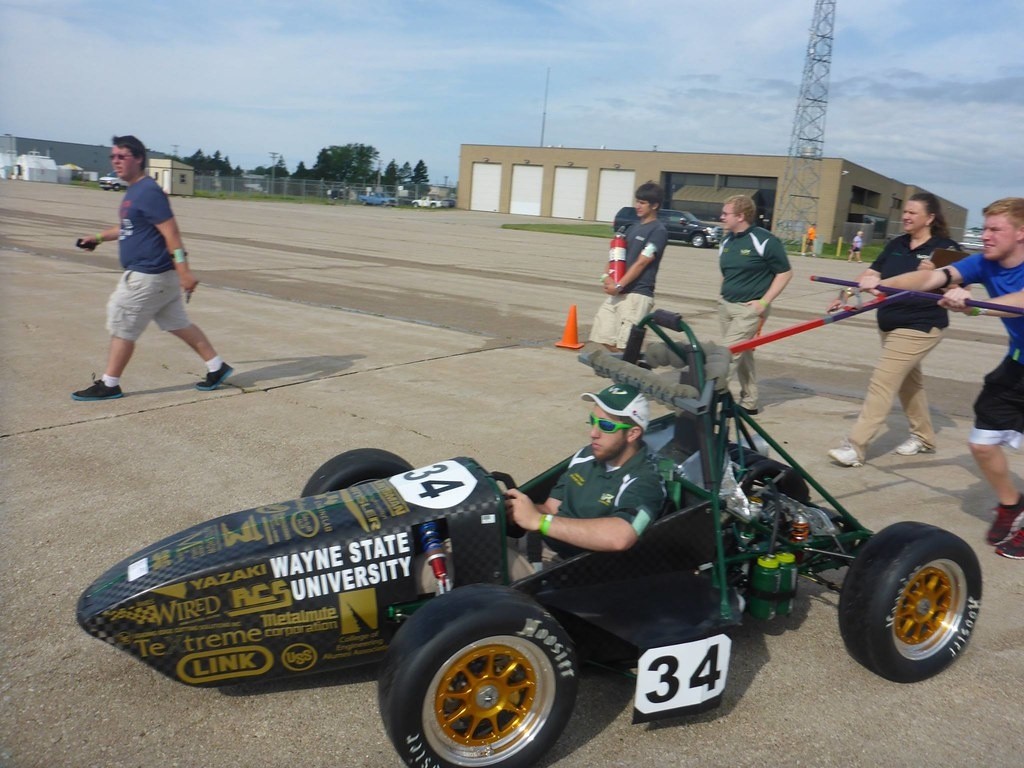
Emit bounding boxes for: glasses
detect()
[589,412,638,434]
[721,211,741,216]
[109,153,136,160]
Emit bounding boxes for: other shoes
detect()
[720,405,758,417]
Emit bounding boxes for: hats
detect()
[580,382,649,433]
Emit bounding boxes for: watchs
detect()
[614,283,624,293]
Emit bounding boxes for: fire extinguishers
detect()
[608,226,627,281]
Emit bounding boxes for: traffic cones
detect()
[554,305,585,350]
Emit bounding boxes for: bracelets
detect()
[95,232,102,244]
[842,286,852,297]
[168,249,187,263]
[938,268,952,289]
[540,512,554,535]
[965,298,987,316]
[599,273,609,283]
[758,299,769,308]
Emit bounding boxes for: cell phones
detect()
[76,238,96,251]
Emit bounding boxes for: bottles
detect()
[747,555,781,620]
[774,552,798,616]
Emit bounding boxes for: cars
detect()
[613,206,724,248]
[356,191,397,207]
[411,194,456,209]
[100,170,129,192]
[327,183,349,198]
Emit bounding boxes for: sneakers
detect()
[71,379,124,401]
[896,433,933,456]
[986,494,1024,546]
[195,362,233,390]
[827,445,865,466]
[995,526,1024,560]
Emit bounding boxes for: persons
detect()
[826,194,972,467]
[859,196,1024,559]
[502,384,667,582]
[591,181,668,368]
[848,229,866,264]
[801,223,819,258]
[716,194,793,416]
[71,134,235,401]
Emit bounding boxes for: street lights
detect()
[271,152,278,193]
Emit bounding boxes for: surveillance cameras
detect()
[841,171,850,176]
